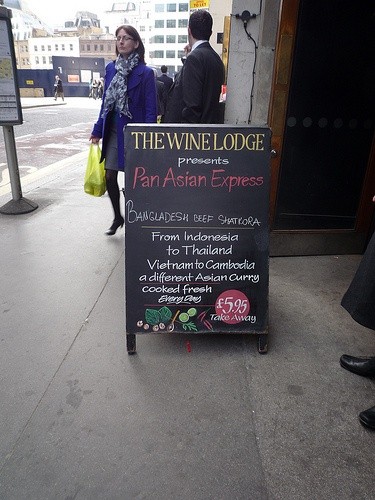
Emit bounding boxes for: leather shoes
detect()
[340,354,375,382]
[358,406,375,431]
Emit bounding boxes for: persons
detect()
[161,9,225,124]
[151,68,164,116]
[339,196,375,432]
[53,74,64,101]
[158,65,173,91]
[89,78,104,101]
[89,25,158,235]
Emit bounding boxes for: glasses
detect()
[114,37,134,41]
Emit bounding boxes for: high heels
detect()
[104,216,124,235]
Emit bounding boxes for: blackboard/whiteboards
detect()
[124,122,271,335]
